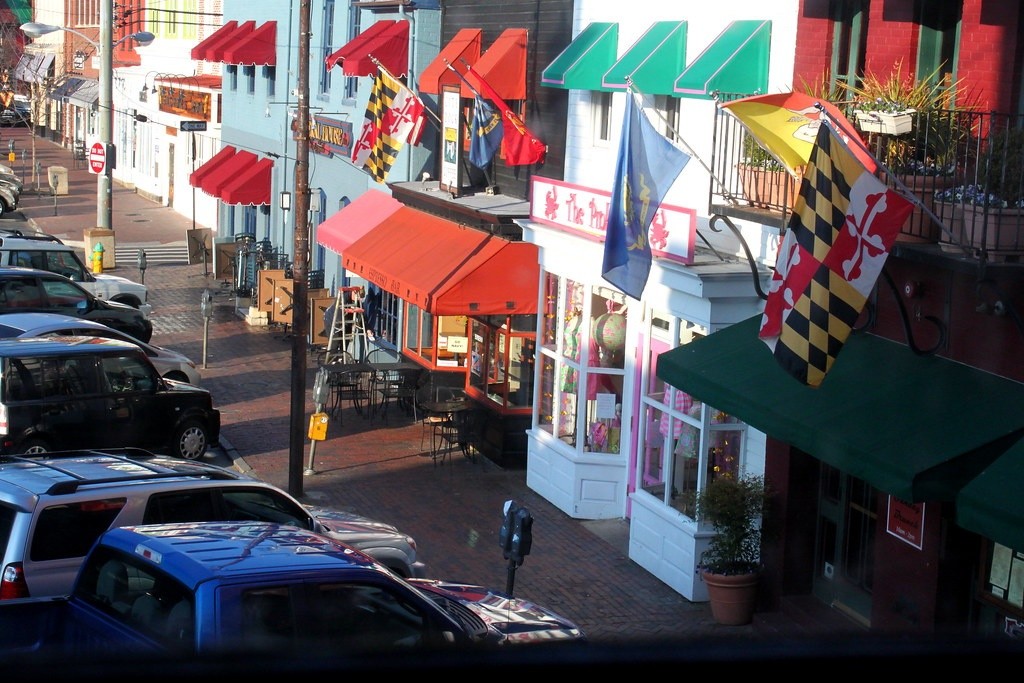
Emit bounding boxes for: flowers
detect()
[856,98,906,112]
[934,185,1024,209]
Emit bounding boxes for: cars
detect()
[0,335,222,461]
[1,94,32,126]
[0,311,202,391]
[0,164,24,218]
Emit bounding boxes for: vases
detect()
[934,200,963,253]
[853,109,917,135]
[957,204,1024,263]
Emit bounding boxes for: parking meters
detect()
[200,289,213,368]
[51,174,58,215]
[8,138,15,168]
[21,149,26,182]
[308,366,331,470]
[36,162,42,198]
[138,248,147,284]
[497,500,534,597]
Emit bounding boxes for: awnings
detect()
[419,27,527,99]
[191,20,277,67]
[541,21,772,103]
[190,146,274,206]
[50,78,99,110]
[657,304,1024,552]
[325,20,408,79]
[315,188,538,316]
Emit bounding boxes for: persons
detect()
[574,315,599,443]
[323,285,375,366]
[678,398,717,495]
[660,383,693,494]
[558,314,582,437]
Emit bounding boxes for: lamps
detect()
[265,102,348,128]
[142,71,199,99]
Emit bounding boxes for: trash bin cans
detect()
[82,227,115,269]
[47,166,68,195]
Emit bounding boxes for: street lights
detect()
[20,21,156,228]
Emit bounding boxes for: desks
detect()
[317,362,424,410]
[421,402,471,457]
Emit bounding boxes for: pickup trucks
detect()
[0,518,588,670]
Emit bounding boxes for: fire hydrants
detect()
[88,243,105,273]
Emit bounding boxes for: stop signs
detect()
[88,141,107,175]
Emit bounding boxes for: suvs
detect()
[0,229,152,316]
[0,446,426,601]
[0,267,153,342]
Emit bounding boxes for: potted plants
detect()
[740,56,959,237]
[679,474,776,625]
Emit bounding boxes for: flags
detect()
[469,94,503,169]
[757,118,916,388]
[352,67,424,183]
[470,66,545,165]
[601,88,692,301]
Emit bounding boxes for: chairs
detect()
[235,232,324,308]
[72,141,88,169]
[316,345,475,465]
